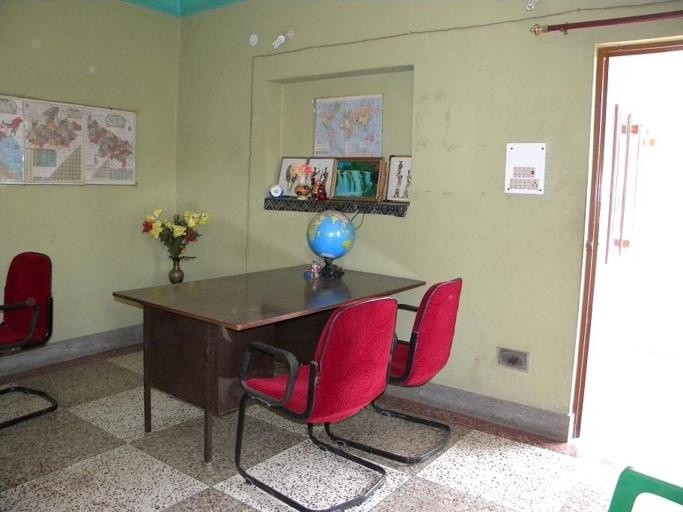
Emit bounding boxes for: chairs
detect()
[322,277,462,465]
[235,295,398,512]
[0,251,58,429]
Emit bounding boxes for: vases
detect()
[168,259,184,283]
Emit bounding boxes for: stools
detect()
[607,465,683,512]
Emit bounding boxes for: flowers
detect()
[140,208,209,261]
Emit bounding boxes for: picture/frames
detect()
[329,157,386,202]
[278,156,335,199]
[385,154,411,204]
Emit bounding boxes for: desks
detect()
[112,263,426,465]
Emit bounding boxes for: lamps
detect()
[272,32,287,49]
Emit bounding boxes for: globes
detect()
[307,209,356,280]
